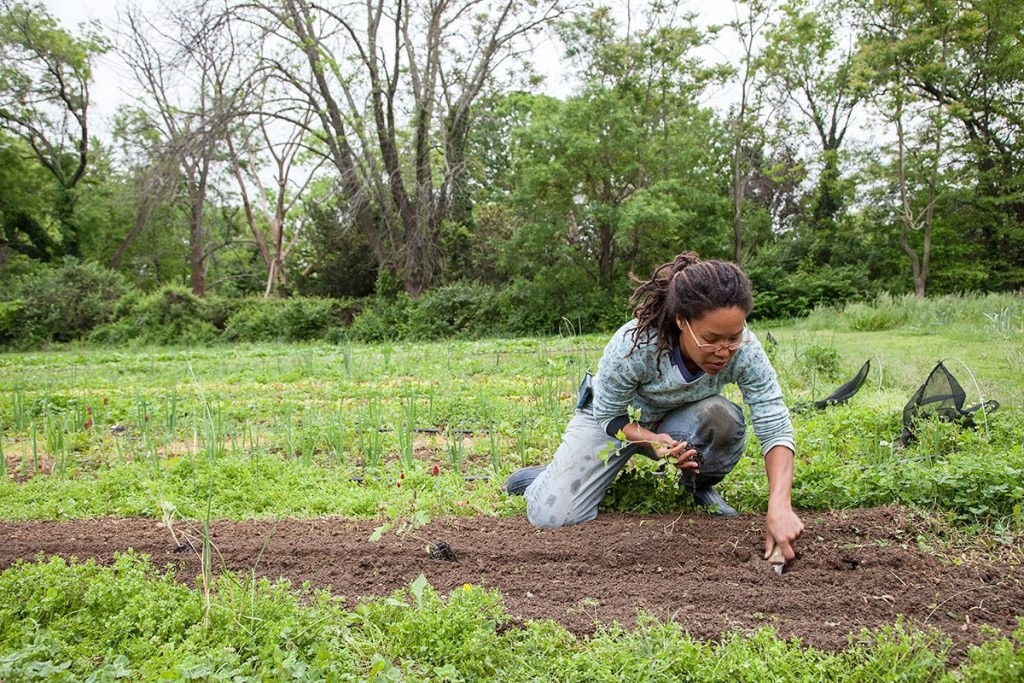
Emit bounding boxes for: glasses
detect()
[684,317,751,353]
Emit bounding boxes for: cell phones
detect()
[576,372,592,410]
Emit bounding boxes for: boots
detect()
[678,469,738,517]
[499,466,546,496]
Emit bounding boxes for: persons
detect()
[505,247,807,560]
